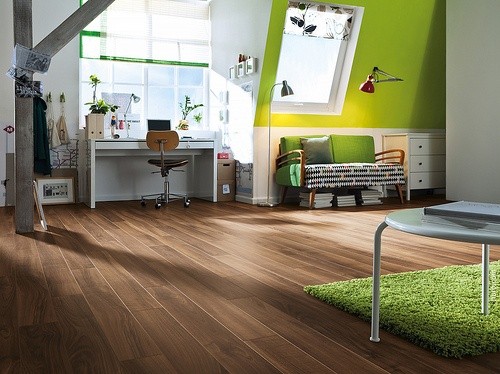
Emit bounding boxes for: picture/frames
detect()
[245,58,256,74]
[228,65,236,79]
[36,177,77,204]
[33,180,48,231]
[220,90,229,107]
[236,61,246,77]
[219,109,229,125]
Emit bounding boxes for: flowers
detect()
[85,74,120,115]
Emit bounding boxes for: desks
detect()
[84,138,218,208]
[370,207,500,342]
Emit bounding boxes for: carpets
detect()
[303,259,500,359]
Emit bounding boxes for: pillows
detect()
[299,136,333,165]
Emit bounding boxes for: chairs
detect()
[140,130,190,209]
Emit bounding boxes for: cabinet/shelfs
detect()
[381,132,445,201]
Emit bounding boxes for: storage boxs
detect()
[217,159,236,180]
[217,180,235,203]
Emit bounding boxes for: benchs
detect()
[275,135,406,210]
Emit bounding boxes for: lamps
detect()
[257,80,295,207]
[124,94,140,138]
[360,67,404,93]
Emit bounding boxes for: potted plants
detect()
[175,95,205,138]
[193,113,203,129]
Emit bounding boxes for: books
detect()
[424,201,500,221]
[334,195,356,206]
[299,192,334,208]
[348,188,384,206]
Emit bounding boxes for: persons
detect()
[111,116,117,138]
[46,186,52,196]
[60,185,68,196]
[52,185,60,196]
[16,69,29,81]
[38,58,48,70]
